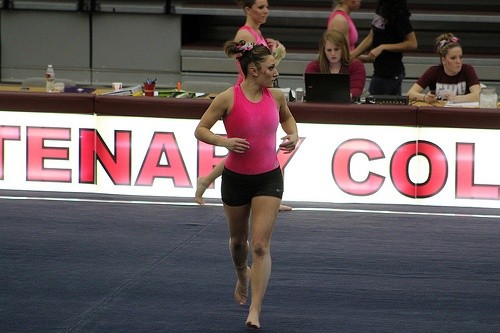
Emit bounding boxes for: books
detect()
[100,86,143,95]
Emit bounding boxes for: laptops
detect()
[304,72,351,103]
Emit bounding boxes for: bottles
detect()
[478,88,498,109]
[46,65,55,92]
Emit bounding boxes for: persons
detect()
[196,0,293,212]
[304,28,366,98]
[405,33,481,103]
[194,40,299,329]
[327,0,372,62]
[349,0,417,96]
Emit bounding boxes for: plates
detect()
[63,88,96,94]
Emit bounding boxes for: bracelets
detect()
[423,95,425,102]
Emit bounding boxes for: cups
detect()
[112,83,123,91]
[143,83,155,97]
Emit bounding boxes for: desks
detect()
[0,82,500,218]
